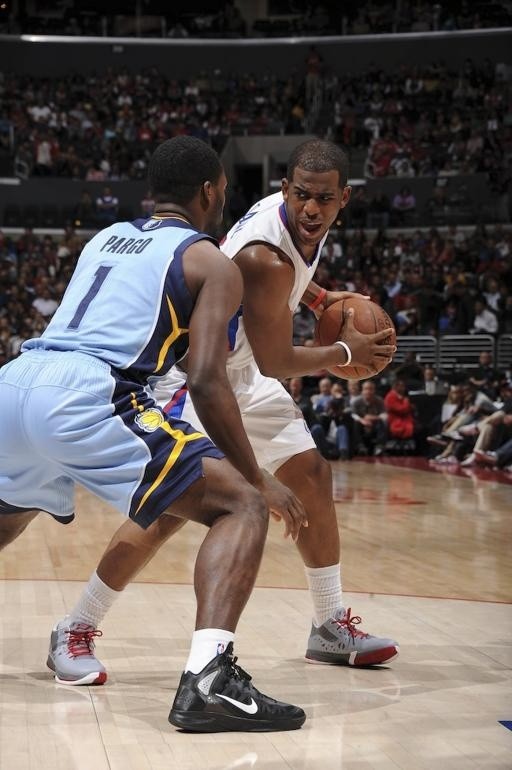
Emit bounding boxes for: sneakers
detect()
[325,446,381,460]
[429,431,499,469]
[308,609,401,670]
[168,642,307,735]
[46,619,108,686]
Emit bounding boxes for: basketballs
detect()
[313,298,396,380]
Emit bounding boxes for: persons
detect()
[1,1,511,180]
[0,134,309,732]
[44,138,401,688]
[1,184,510,471]
